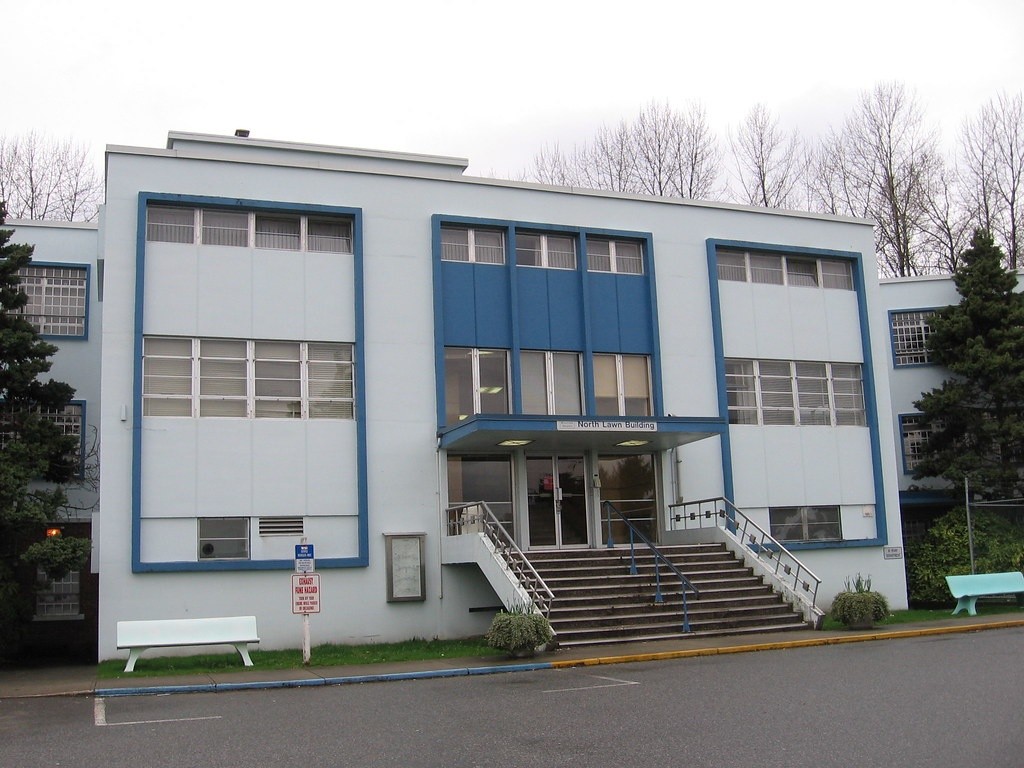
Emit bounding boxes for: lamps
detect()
[121,404,126,421]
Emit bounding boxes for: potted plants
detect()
[484,590,554,657]
[831,572,890,630]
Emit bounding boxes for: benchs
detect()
[945,572,1024,616]
[116,616,261,672]
